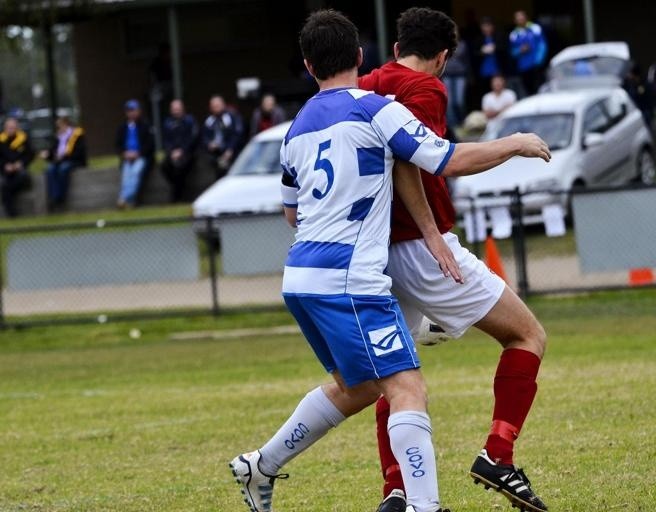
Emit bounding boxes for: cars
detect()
[191,119,299,219]
[442,41,653,234]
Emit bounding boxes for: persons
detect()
[440,12,546,129]
[39,115,88,214]
[352,7,551,511]
[248,93,286,141]
[159,99,198,204]
[228,10,549,512]
[1,116,34,218]
[116,99,153,208]
[199,95,243,179]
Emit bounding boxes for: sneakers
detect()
[375,489,405,511]
[230,450,279,512]
[470,447,549,512]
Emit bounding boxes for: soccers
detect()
[416,315,450,346]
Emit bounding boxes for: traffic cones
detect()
[485,238,506,287]
[625,268,656,284]
